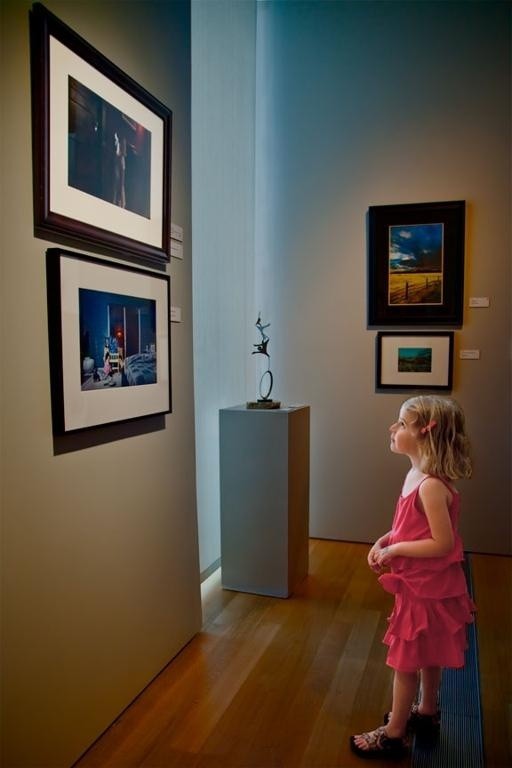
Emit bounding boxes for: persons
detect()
[102,352,113,380]
[350,396,479,758]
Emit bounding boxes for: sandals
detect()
[350,726,409,758]
[384,705,441,730]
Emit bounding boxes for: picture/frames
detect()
[30,2,174,264]
[365,199,467,389]
[44,247,174,437]
